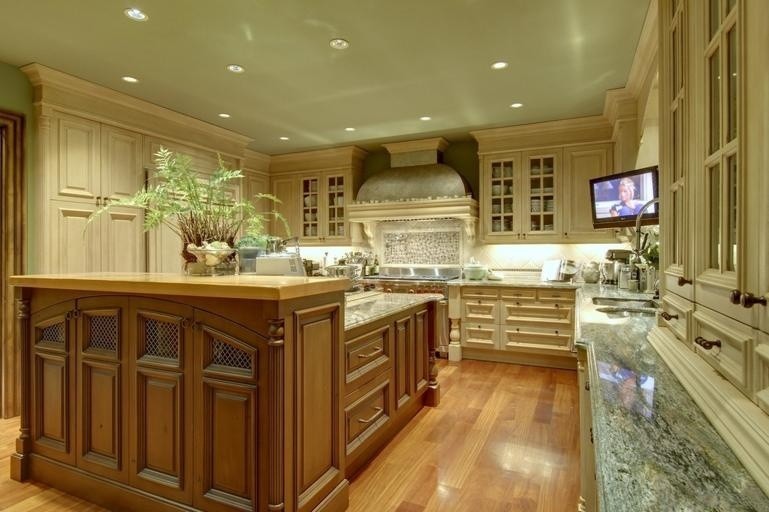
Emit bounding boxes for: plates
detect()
[305,214,316,221]
[492,199,554,231]
[337,225,344,236]
[304,227,317,237]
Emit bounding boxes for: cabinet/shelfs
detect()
[345,301,428,487]
[241,148,270,237]
[6,285,351,511]
[297,146,371,247]
[561,115,622,245]
[16,61,256,275]
[467,119,562,246]
[460,286,577,372]
[269,151,298,247]
[692,1,769,502]
[645,0,690,395]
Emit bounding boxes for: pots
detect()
[266,236,299,253]
[326,260,355,279]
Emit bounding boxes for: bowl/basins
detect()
[492,184,512,195]
[532,187,553,194]
[463,264,486,281]
[560,264,577,280]
[239,247,259,258]
[187,248,235,266]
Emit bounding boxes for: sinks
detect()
[596,308,657,316]
[593,296,660,308]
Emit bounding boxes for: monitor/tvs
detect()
[595,360,656,421]
[589,165,659,229]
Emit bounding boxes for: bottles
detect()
[620,262,655,292]
[581,262,600,284]
[348,251,380,277]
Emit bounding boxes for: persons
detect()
[611,366,647,401]
[609,177,648,217]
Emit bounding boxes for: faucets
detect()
[654,279,659,292]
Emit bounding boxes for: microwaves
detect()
[366,274,459,293]
